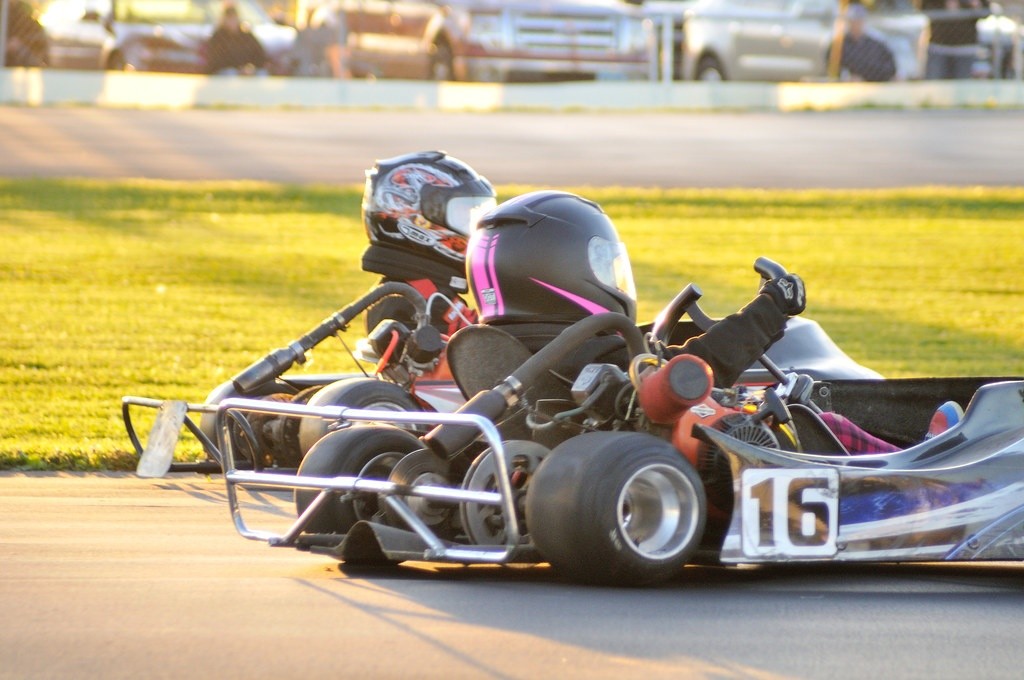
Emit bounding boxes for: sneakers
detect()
[922,400,964,445]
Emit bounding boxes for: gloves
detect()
[758,271,805,317]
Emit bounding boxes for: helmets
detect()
[360,150,498,293]
[465,189,640,340]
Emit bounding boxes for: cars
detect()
[682,0,1016,85]
[34,0,299,74]
[313,0,657,86]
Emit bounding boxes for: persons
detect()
[208,7,268,76]
[360,150,497,379]
[465,191,964,456]
[826,7,896,83]
[914,0,991,79]
[5,2,49,67]
